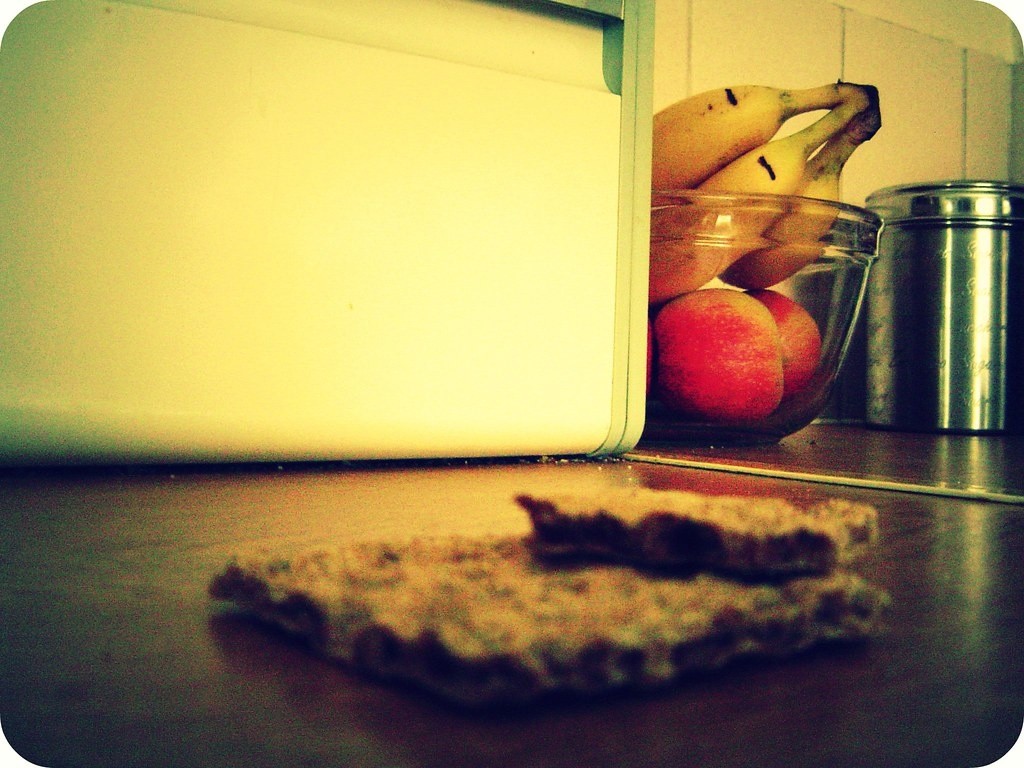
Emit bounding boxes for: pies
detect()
[209,488,894,708]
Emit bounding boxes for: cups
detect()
[868,180,1024,435]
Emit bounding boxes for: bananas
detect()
[649,82,880,301]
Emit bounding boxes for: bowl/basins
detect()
[651,186,886,447]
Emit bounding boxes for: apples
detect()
[647,290,821,423]
